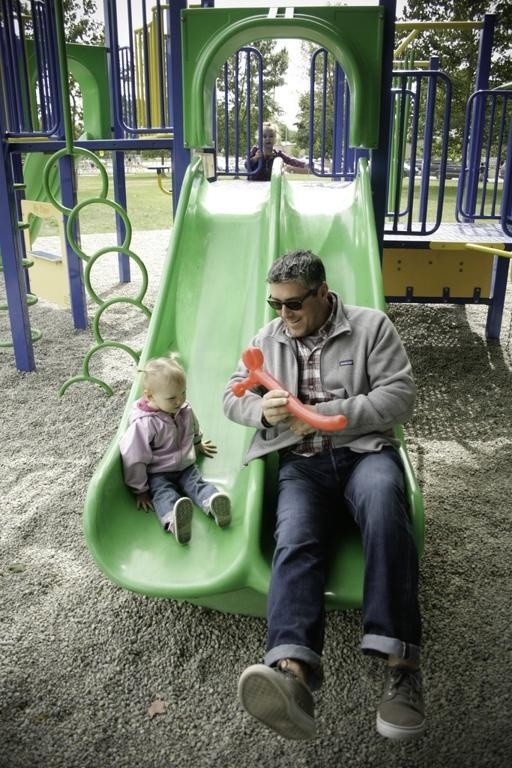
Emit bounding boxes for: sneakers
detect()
[236,657,317,742]
[208,491,232,528]
[375,662,428,741]
[168,496,194,545]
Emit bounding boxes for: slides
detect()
[82,154,424,616]
[21,151,77,244]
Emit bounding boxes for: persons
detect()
[222,253,427,742]
[118,357,231,543]
[244,118,315,182]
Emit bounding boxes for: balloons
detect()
[234,347,348,432]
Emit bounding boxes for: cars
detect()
[404,159,461,180]
[499,158,506,177]
[283,156,329,174]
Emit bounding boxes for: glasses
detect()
[266,289,312,311]
